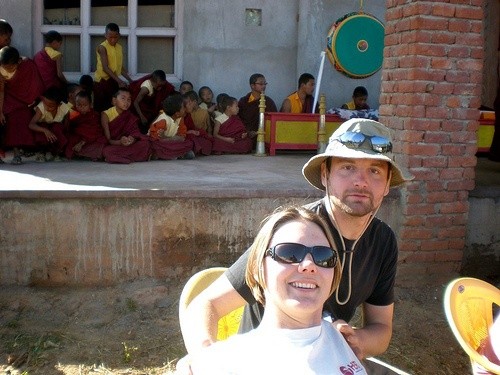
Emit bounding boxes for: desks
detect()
[265,109,496,157]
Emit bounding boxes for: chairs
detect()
[178,267,412,375]
[442,276,500,375]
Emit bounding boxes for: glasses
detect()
[255,82,268,86]
[264,242,338,268]
[329,131,393,153]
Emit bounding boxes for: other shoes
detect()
[11,151,22,164]
[35,152,48,162]
[179,151,196,160]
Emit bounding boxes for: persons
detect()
[473,314,500,375]
[101,88,152,163]
[128,70,166,131]
[33,32,63,89]
[209,206,368,375]
[29,76,104,161]
[147,96,195,160]
[0,19,42,164]
[213,98,253,154]
[182,118,415,375]
[238,74,278,149]
[180,81,193,95]
[341,86,369,110]
[184,90,212,155]
[279,73,321,114]
[197,86,229,132]
[93,25,133,113]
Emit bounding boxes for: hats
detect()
[301,117,415,192]
